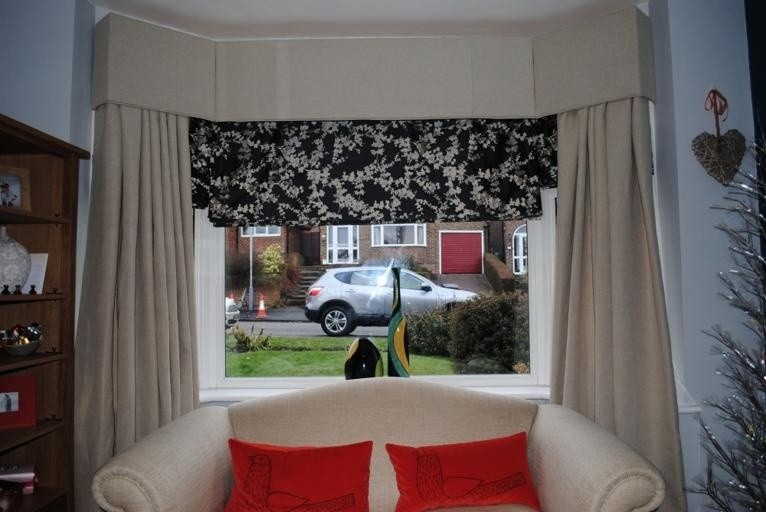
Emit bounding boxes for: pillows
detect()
[384,432,541,512]
[219,431,376,512]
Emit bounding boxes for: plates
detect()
[1,339,41,356]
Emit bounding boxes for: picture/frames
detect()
[0,373,39,429]
[1,162,32,214]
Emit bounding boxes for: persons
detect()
[4,393,12,413]
[0,182,18,208]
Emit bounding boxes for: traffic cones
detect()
[256,292,267,319]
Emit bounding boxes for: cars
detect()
[305,266,481,337]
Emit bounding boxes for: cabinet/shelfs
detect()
[0,113,91,511]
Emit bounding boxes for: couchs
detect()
[91,376,668,512]
